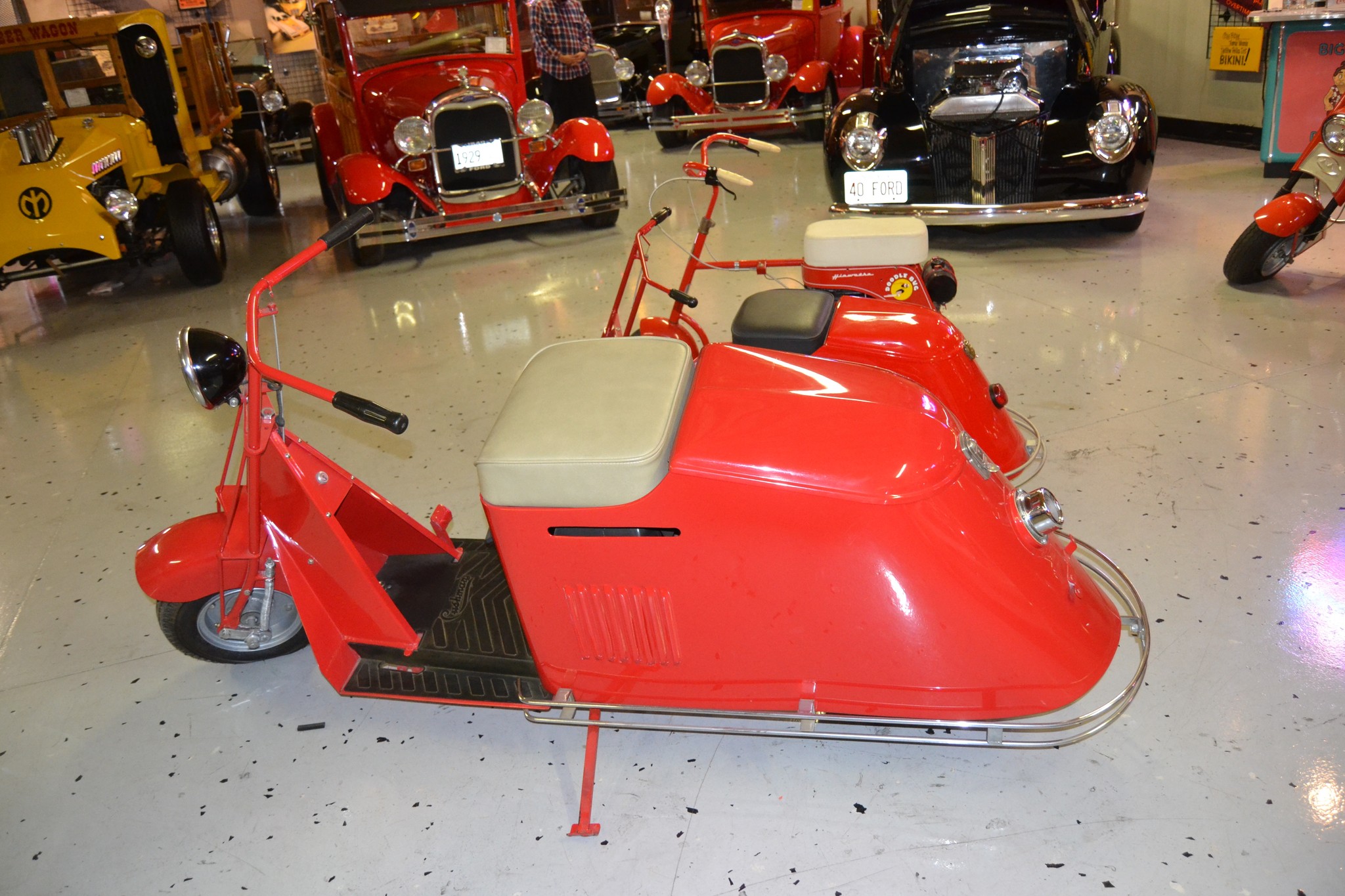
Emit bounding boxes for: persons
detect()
[528,0,600,128]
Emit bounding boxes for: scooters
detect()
[1221,90,1345,286]
[616,130,959,353]
[132,201,1152,752]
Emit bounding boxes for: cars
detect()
[643,0,879,151]
[0,11,283,292]
[303,0,631,272]
[522,17,709,129]
[819,0,1159,244]
[219,62,317,167]
